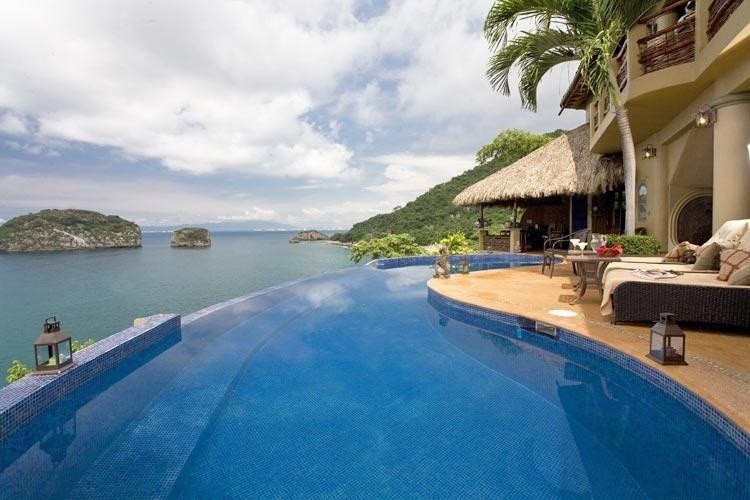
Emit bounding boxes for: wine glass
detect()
[577,242,588,256]
[570,239,581,252]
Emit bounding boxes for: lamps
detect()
[694,103,716,128]
[642,144,656,160]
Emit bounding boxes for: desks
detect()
[566,248,622,306]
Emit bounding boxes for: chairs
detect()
[542,229,592,280]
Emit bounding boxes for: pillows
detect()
[662,237,750,290]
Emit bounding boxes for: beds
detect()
[599,218,750,333]
